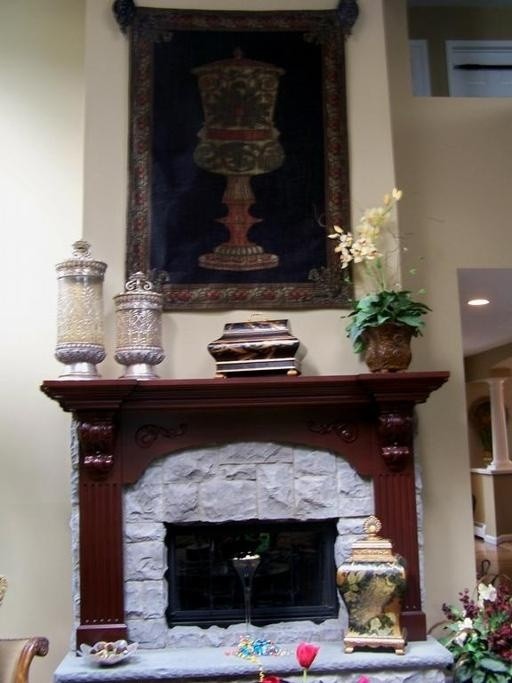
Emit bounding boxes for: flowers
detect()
[426,558,512,683]
[326,183,435,355]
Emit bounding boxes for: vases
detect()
[359,320,414,375]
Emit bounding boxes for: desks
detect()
[52,634,455,683]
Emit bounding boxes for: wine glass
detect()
[226,551,281,655]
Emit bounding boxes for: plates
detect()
[72,638,140,665]
